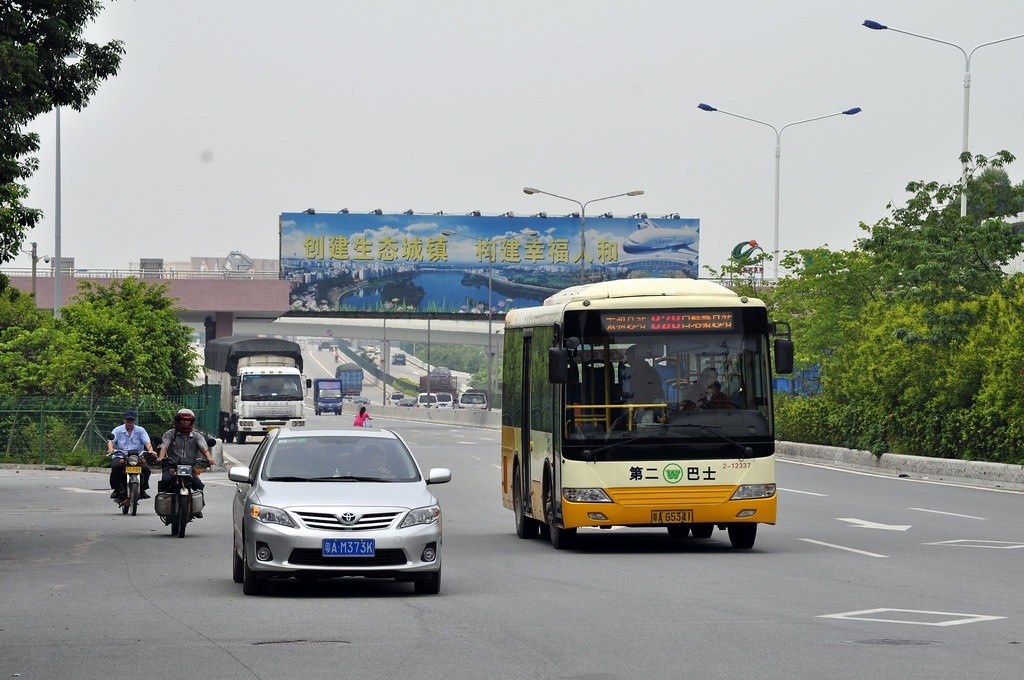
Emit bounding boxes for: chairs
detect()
[568,358,744,413]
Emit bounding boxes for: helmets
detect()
[174,409,195,433]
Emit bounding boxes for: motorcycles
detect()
[105,432,159,517]
[153,436,217,538]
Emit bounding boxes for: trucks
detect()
[203,333,312,445]
[336,363,364,398]
[312,378,346,416]
[416,375,459,404]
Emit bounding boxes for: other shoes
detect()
[111,491,117,498]
[195,511,203,518]
[141,491,151,498]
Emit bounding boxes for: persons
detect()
[107,412,158,499]
[625,345,665,415]
[335,344,339,351]
[156,409,216,527]
[344,445,394,479]
[353,407,373,427]
[282,377,299,392]
[319,343,322,351]
[681,367,738,412]
[335,352,339,360]
[330,344,333,352]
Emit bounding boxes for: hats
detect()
[124,411,137,421]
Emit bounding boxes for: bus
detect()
[501,279,794,551]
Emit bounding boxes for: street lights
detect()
[862,19,1024,217]
[442,229,539,394]
[522,187,645,284]
[53,53,79,319]
[698,102,864,285]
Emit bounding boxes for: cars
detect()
[387,388,488,412]
[318,341,336,352]
[432,366,452,375]
[362,344,381,355]
[391,352,406,366]
[227,426,453,597]
